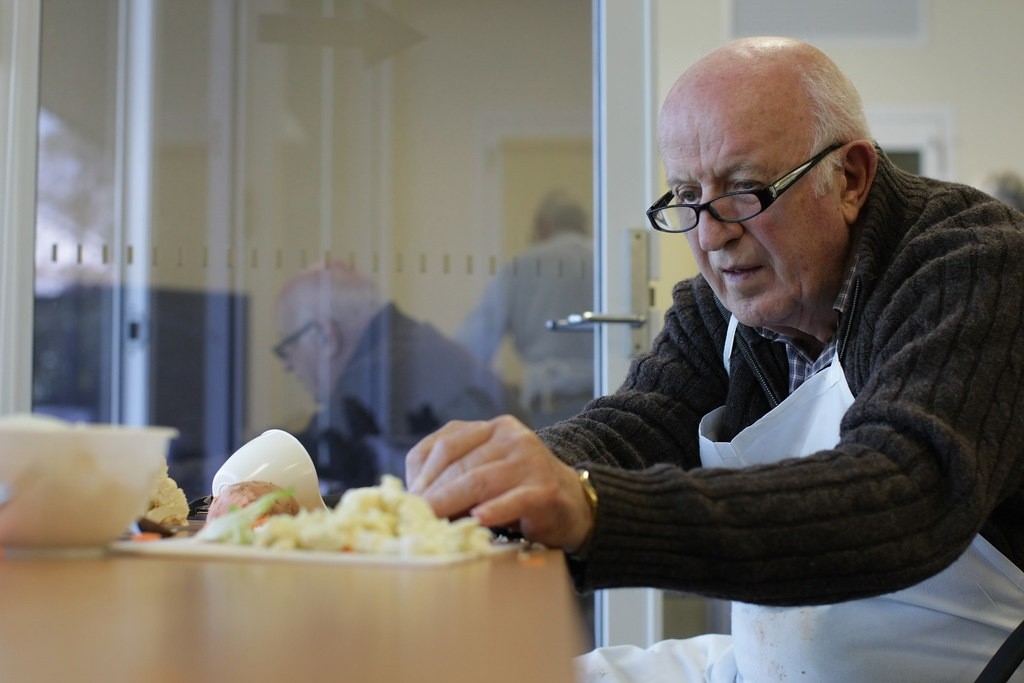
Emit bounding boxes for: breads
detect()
[137,463,189,533]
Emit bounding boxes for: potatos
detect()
[206,481,299,526]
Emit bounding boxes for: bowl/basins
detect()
[0,425,182,551]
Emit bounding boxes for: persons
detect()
[274,263,534,504]
[458,192,598,418]
[405,35,1024,683]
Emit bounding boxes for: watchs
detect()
[577,466,598,507]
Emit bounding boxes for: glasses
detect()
[645,140,848,235]
[270,318,315,362]
